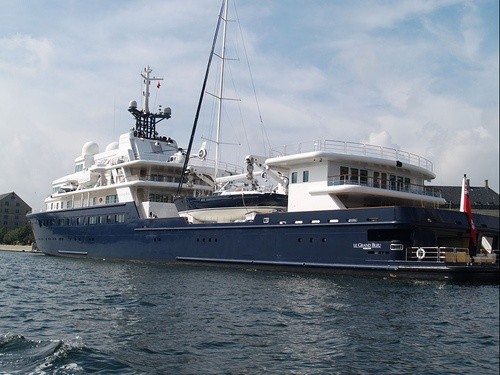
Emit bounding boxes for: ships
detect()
[23,0,500,290]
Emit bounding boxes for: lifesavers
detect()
[416,248,425,259]
